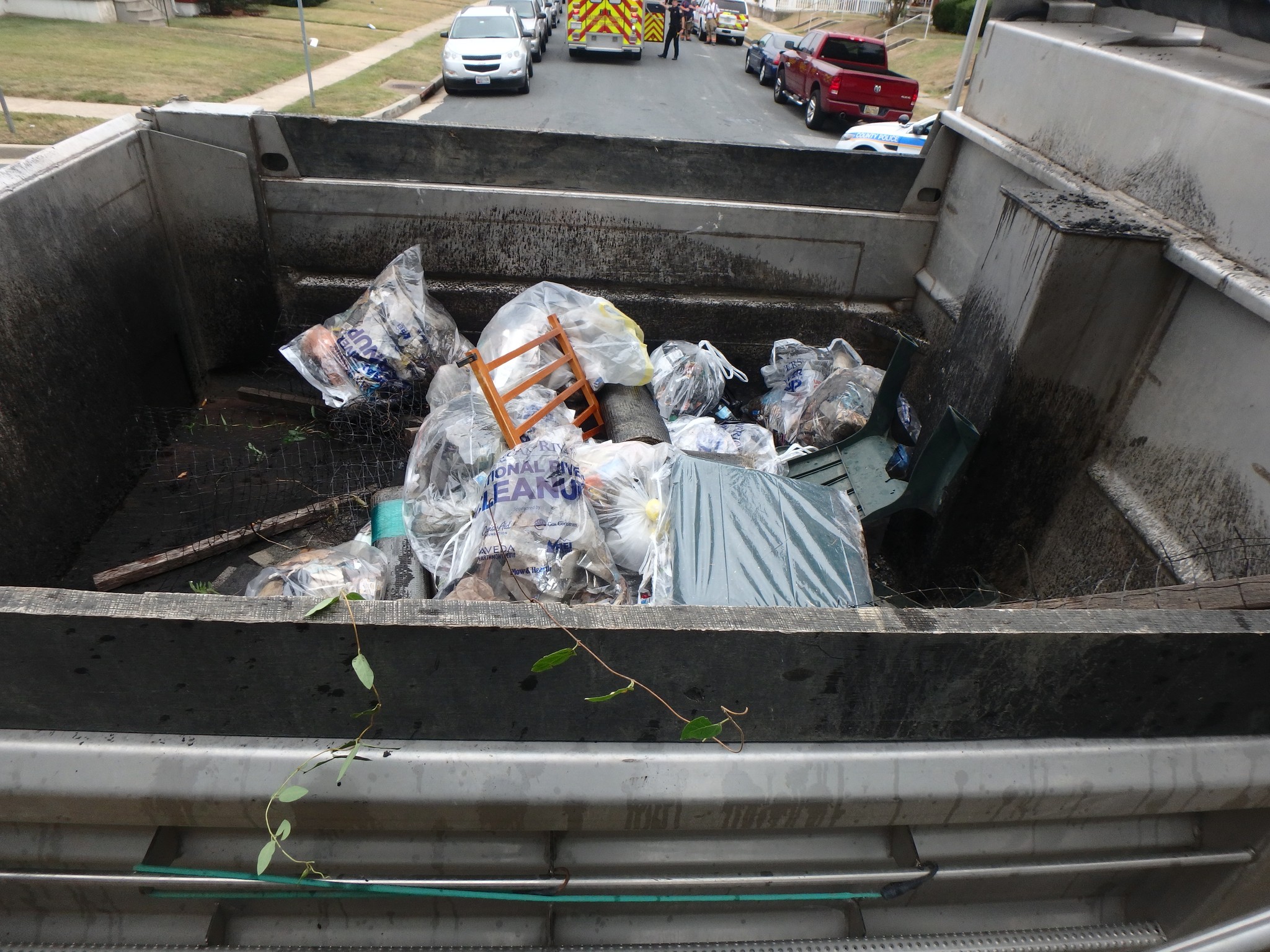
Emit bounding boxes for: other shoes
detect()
[686,39,691,41]
[712,43,716,45]
[680,38,684,40]
[704,41,711,44]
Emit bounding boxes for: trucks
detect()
[1,2,1270,952]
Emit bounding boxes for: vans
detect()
[565,0,666,60]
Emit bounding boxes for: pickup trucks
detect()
[774,29,919,135]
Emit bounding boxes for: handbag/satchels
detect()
[242,242,889,612]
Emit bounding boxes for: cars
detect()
[835,106,963,154]
[440,4,533,95]
[487,0,562,63]
[691,0,749,46]
[746,32,805,86]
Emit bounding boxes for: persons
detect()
[704,0,720,46]
[680,0,696,42]
[657,0,685,60]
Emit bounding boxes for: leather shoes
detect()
[658,54,666,58]
[672,57,677,60]
